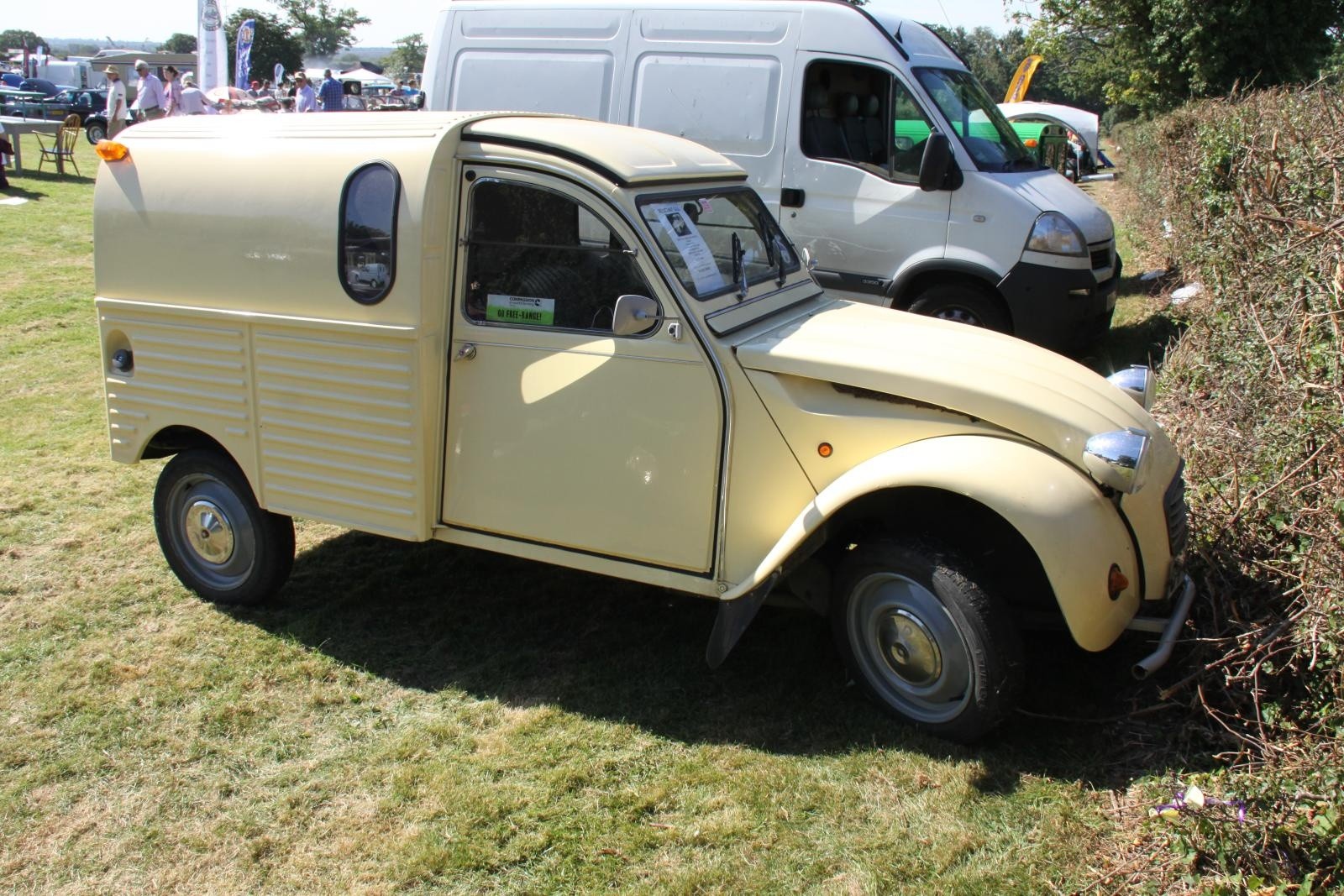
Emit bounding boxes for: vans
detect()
[420,1,1124,348]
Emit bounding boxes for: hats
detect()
[180,73,194,84]
[323,69,332,76]
[251,81,259,88]
[103,65,121,74]
[294,71,306,79]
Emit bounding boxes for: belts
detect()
[137,105,160,112]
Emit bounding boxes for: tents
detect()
[339,68,393,85]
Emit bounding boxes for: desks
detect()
[0,90,47,122]
[0,115,65,176]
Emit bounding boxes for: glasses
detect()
[296,79,306,83]
[136,68,147,72]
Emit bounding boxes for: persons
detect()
[181,82,215,114]
[258,79,272,96]
[246,81,259,96]
[134,59,167,120]
[319,68,344,110]
[294,71,316,112]
[102,64,127,141]
[409,80,417,88]
[275,82,286,109]
[288,82,296,96]
[163,65,185,117]
[389,79,404,104]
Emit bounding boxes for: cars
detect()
[1,62,418,147]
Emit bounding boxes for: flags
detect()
[1003,55,1042,124]
[198,0,229,104]
[235,19,255,89]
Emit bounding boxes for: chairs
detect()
[80,97,89,108]
[803,78,886,162]
[32,114,81,176]
[516,256,650,330]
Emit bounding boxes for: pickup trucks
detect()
[91,110,1199,752]
